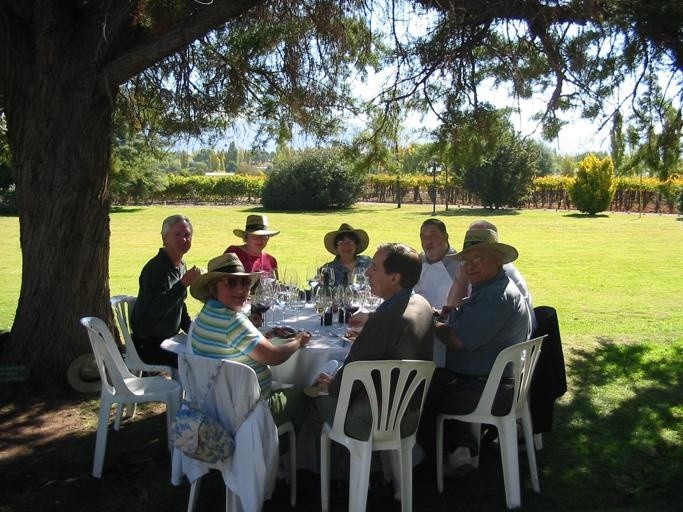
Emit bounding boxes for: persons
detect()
[316,223,371,294]
[300,241,436,493]
[225,215,279,294]
[412,218,473,319]
[128,215,200,370]
[188,254,311,468]
[445,219,532,312]
[419,220,537,479]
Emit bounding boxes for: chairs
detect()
[108,294,181,432]
[317,358,438,512]
[174,351,299,512]
[78,315,184,486]
[433,333,551,508]
[467,306,559,468]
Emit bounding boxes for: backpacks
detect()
[172,352,263,463]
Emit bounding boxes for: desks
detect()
[158,298,447,472]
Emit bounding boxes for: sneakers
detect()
[448,446,473,471]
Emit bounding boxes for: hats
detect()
[324,223,369,254]
[190,253,265,300]
[445,228,518,264]
[233,215,280,238]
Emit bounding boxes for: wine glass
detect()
[253,267,307,330]
[307,267,382,333]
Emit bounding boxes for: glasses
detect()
[336,240,355,245]
[216,276,251,288]
[461,254,499,265]
